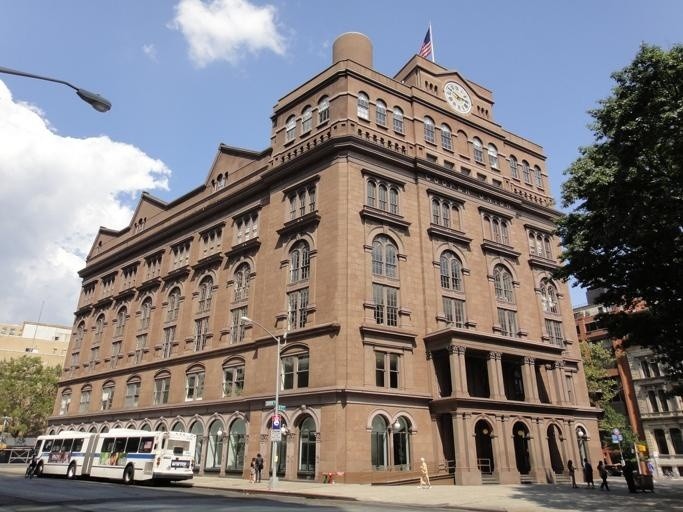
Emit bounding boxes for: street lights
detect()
[240,312,288,490]
[0,67,111,114]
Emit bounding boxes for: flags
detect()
[419,28,431,57]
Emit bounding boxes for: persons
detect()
[419,457,431,489]
[255,454,263,481]
[621,461,636,493]
[646,458,654,474]
[25,454,42,477]
[597,461,609,491]
[583,458,594,488]
[249,458,259,483]
[567,460,579,488]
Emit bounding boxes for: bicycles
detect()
[24,459,36,478]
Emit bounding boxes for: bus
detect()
[33,428,196,483]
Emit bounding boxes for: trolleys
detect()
[630,473,654,493]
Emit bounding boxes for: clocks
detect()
[444,81,472,114]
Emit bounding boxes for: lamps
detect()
[281,424,292,439]
[575,428,583,437]
[217,428,227,438]
[390,416,400,431]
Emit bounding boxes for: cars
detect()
[604,462,622,476]
